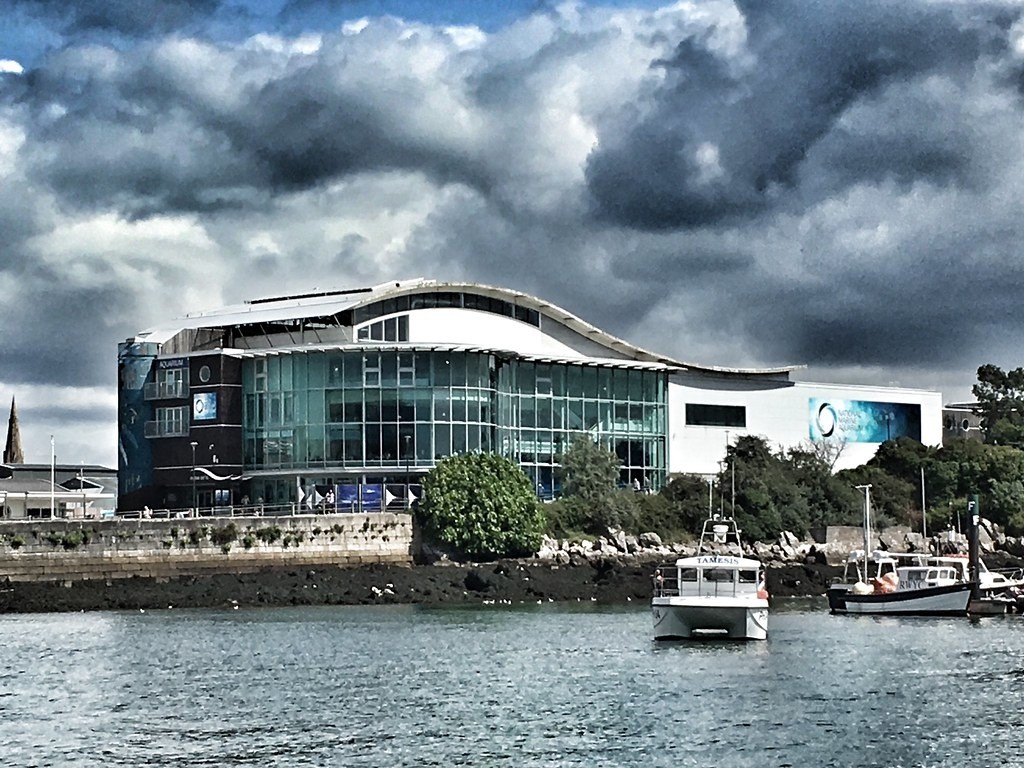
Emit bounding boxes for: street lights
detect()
[191,442,198,517]
[403,435,411,511]
[51,435,56,520]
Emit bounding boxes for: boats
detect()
[650,461,769,640]
[826,466,1016,616]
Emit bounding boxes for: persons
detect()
[241,495,250,513]
[258,497,263,512]
